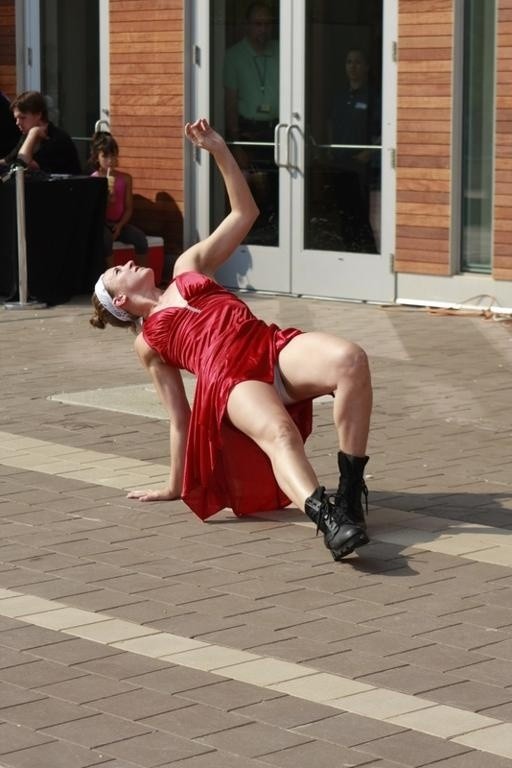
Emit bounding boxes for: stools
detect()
[103,235,163,287]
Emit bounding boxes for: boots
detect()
[304,451,370,561]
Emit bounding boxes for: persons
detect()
[316,44,378,248]
[1,96,22,159]
[88,131,147,268]
[0,91,84,172]
[89,117,374,562]
[221,2,279,244]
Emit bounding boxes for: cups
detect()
[105,176,115,195]
